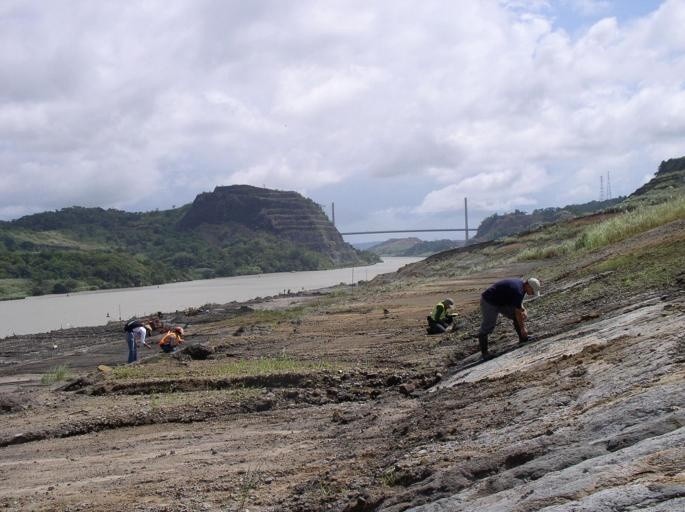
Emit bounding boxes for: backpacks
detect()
[123,320,143,332]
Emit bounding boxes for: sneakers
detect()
[426,327,432,335]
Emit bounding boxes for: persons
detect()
[425,298,460,334]
[123,323,152,364]
[159,326,189,354]
[478,277,541,361]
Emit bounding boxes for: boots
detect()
[512,320,538,343]
[477,332,501,360]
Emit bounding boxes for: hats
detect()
[144,325,152,331]
[443,298,455,309]
[527,277,542,297]
[174,326,185,336]
